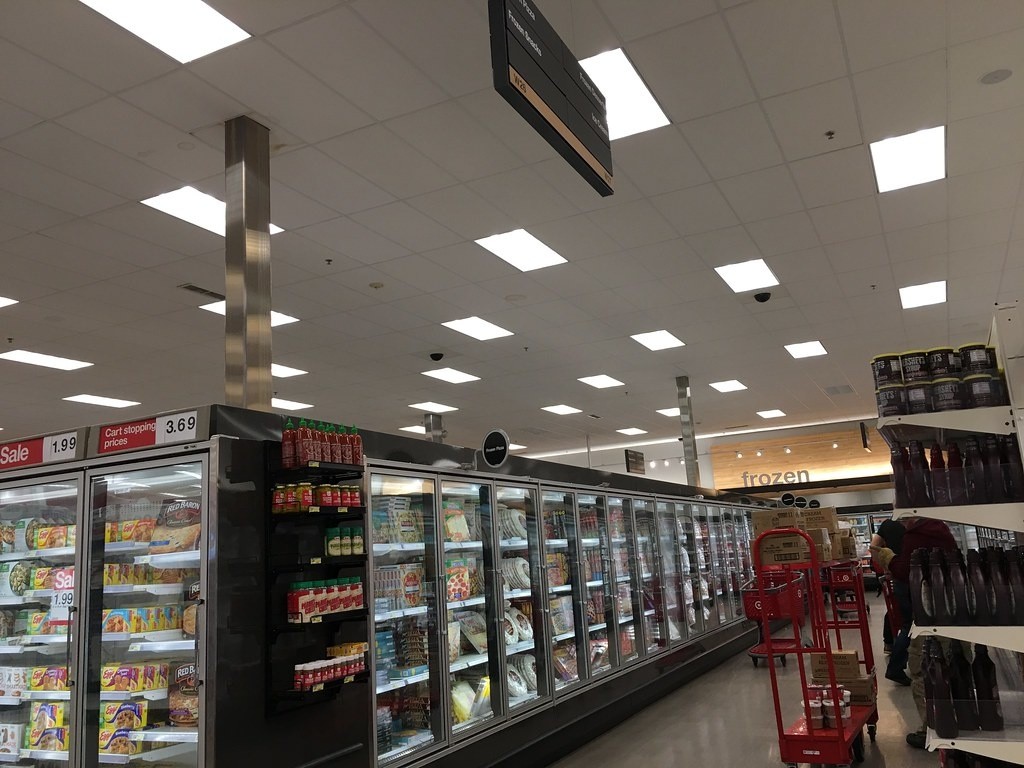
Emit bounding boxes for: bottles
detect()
[287,577,363,623]
[890,434,1024,507]
[282,417,363,468]
[294,653,366,691]
[910,546,1024,625]
[921,640,1002,738]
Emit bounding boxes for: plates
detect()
[505,654,537,697]
[501,557,531,589]
[498,509,527,539]
[504,607,533,645]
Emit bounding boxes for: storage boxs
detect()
[0,517,200,755]
[800,650,873,730]
[752,506,857,565]
[373,496,634,756]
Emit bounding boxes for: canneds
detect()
[869,342,1009,417]
[271,483,364,556]
[293,653,365,691]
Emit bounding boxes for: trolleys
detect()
[741,570,813,666]
[827,562,870,619]
[752,527,880,768]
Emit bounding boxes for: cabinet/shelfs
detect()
[273,461,368,697]
[877,301,1024,766]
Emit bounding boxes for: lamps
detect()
[650,441,839,469]
